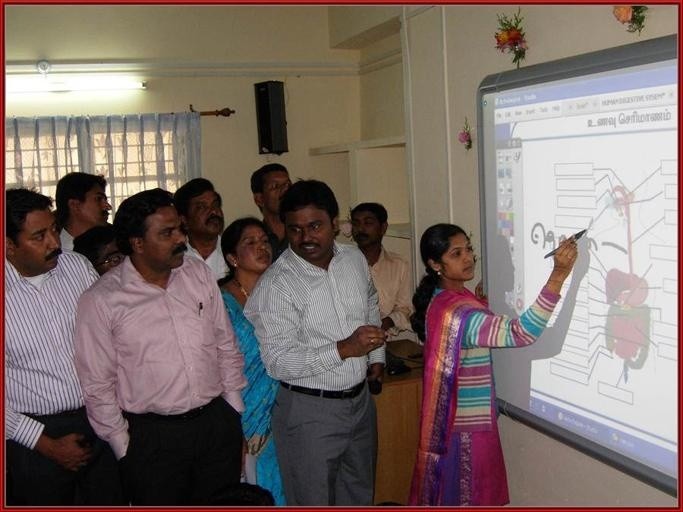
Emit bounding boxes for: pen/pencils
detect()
[544,230,586,258]
[198,303,203,316]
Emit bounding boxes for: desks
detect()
[369,354,424,507]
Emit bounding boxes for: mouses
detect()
[389,364,406,376]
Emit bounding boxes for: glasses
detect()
[96,253,126,267]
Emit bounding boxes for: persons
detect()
[410,224,578,507]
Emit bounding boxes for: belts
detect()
[280,379,366,399]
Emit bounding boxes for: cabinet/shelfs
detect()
[307,139,412,264]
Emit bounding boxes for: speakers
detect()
[253,80,289,154]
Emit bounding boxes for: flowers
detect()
[457,116,473,151]
[492,6,528,70]
[340,204,352,234]
[611,5,647,37]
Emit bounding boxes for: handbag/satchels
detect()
[385,339,424,369]
